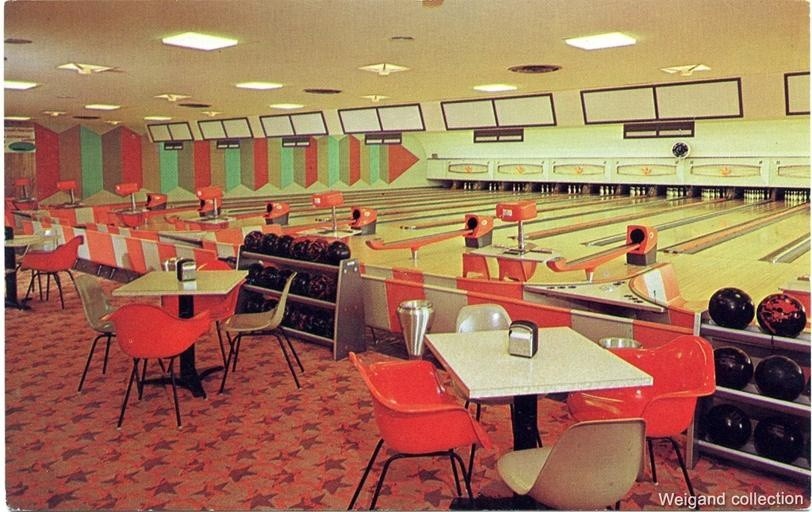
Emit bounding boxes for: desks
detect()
[4,235,61,310]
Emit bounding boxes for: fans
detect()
[671,141,690,159]
